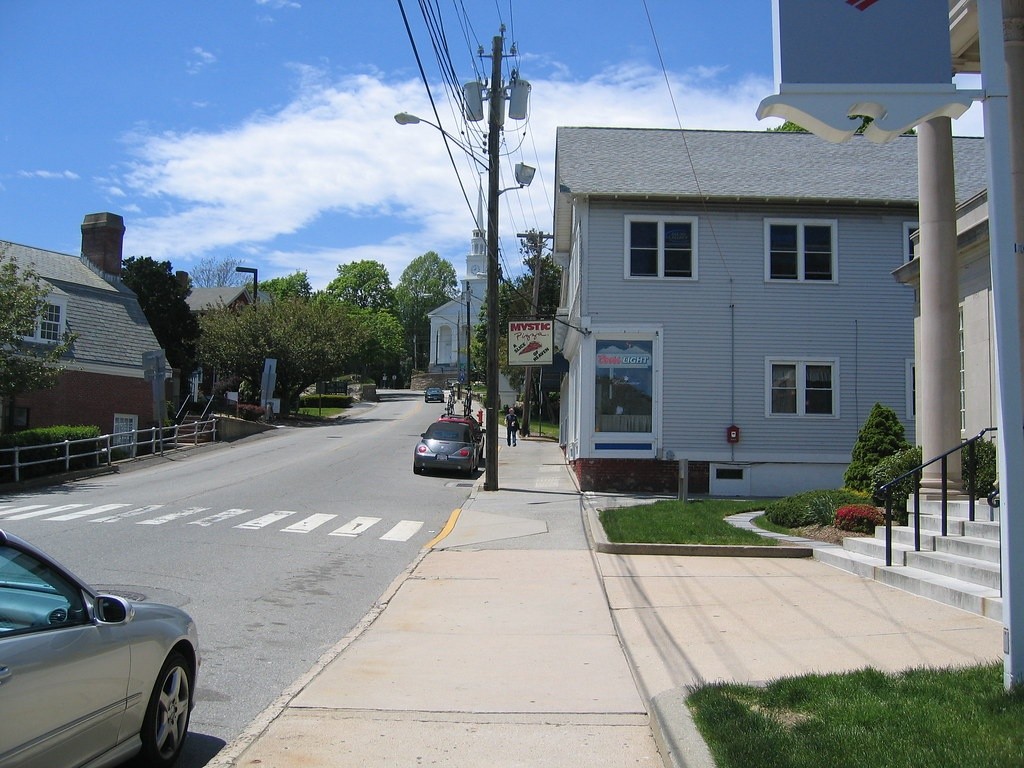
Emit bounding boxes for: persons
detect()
[504,408,518,447]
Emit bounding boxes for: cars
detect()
[413,423,479,476]
[437,412,486,459]
[0,528,202,768]
[423,388,445,403]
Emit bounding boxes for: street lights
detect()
[394,35,535,492]
[422,288,473,411]
[427,310,461,390]
